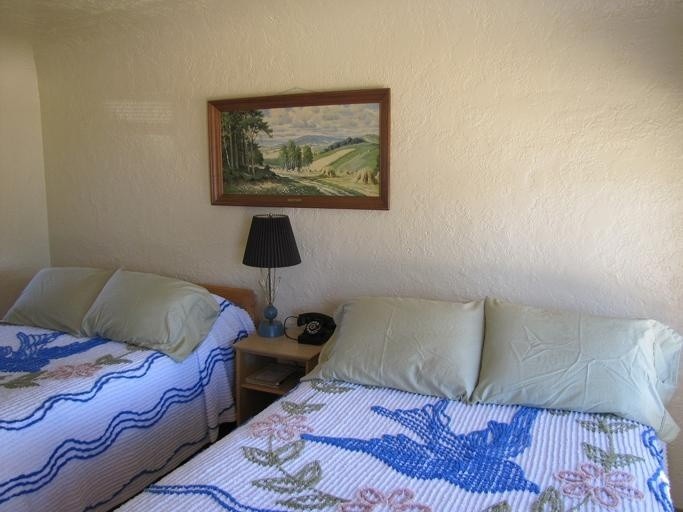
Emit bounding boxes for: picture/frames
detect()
[204,87,393,210]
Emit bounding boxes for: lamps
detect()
[241,213,302,338]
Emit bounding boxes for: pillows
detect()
[0,263,223,363]
[301,296,485,402]
[472,297,681,447]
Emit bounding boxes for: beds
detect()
[112,362,676,512]
[0,284,258,512]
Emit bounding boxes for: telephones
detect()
[297,312,336,346]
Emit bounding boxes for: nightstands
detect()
[234,326,325,425]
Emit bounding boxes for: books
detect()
[245,363,296,389]
